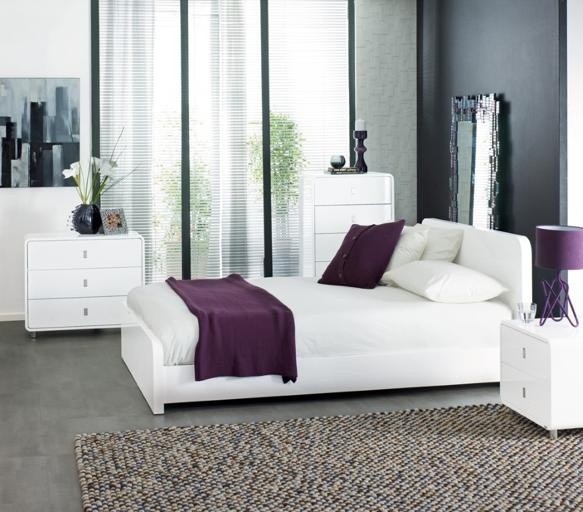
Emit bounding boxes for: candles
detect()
[355,119,366,130]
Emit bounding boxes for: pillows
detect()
[413,223,464,262]
[380,225,429,289]
[318,220,405,289]
[381,260,508,303]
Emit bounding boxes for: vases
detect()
[72,205,100,234]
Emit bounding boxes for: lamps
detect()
[535,225,583,327]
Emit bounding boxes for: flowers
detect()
[62,125,139,204]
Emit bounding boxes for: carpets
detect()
[75,405,583,511]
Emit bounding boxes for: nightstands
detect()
[498,317,583,439]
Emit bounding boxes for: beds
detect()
[120,216,534,416]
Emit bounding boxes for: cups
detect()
[518,302,537,323]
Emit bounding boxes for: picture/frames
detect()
[100,208,128,235]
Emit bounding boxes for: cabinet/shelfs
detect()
[300,172,395,279]
[23,231,145,339]
